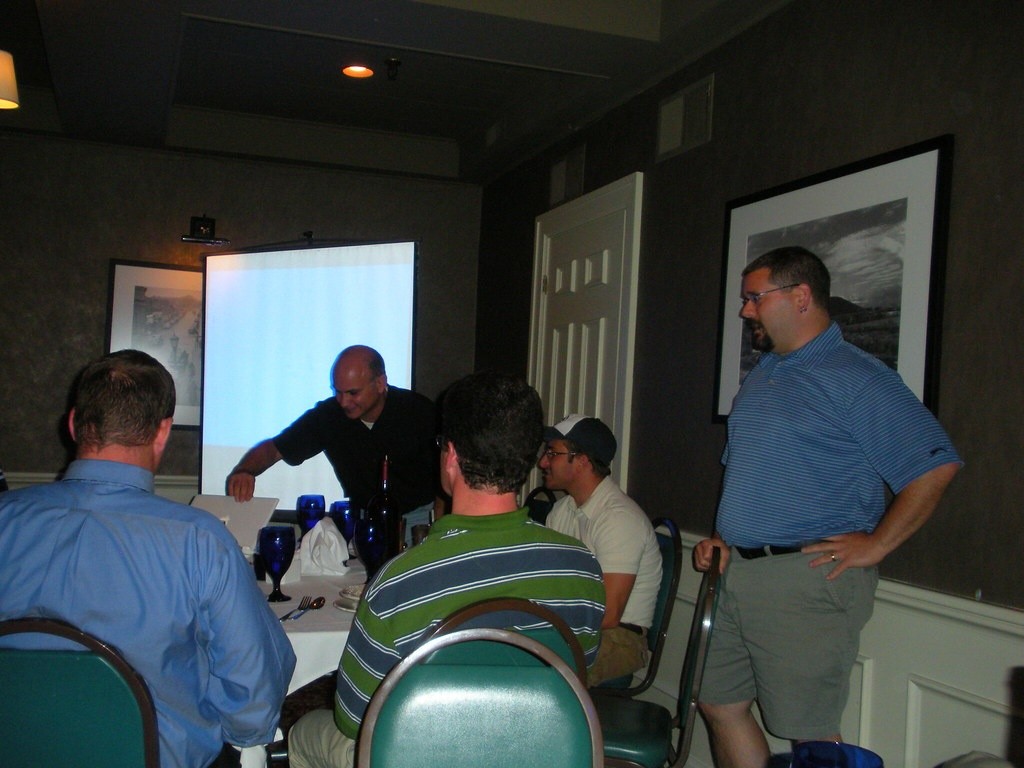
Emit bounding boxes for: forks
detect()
[279,596,311,623]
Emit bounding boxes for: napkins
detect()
[299,516,352,576]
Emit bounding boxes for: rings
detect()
[831,553,836,562]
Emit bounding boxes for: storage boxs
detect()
[266,558,301,584]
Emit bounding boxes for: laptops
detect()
[189,493,279,559]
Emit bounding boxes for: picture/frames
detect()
[104,258,203,431]
[706,134,955,425]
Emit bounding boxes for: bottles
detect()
[369,453,400,558]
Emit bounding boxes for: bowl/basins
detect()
[338,590,360,610]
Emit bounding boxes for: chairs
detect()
[589,515,682,698]
[523,486,557,526]
[594,546,720,768]
[426,598,588,689]
[355,628,604,768]
[0,617,159,768]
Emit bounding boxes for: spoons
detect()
[292,597,325,620]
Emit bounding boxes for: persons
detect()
[693,246,965,768]
[540,413,663,687]
[229,345,452,575]
[0,349,297,768]
[287,366,607,768]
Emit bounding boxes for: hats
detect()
[541,412,617,467]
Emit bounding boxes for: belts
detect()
[735,544,808,559]
[618,622,650,636]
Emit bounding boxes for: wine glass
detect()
[295,496,357,568]
[354,506,379,582]
[259,526,295,603]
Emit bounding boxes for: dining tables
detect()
[255,557,368,697]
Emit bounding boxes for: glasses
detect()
[542,450,577,459]
[436,434,448,451]
[743,284,800,304]
